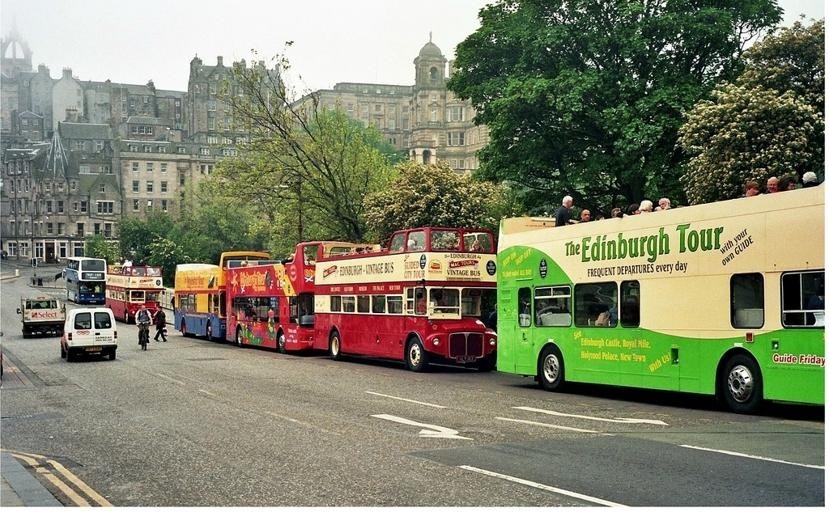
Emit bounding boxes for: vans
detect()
[60,306,118,362]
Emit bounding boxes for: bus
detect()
[62,256,107,304]
[494,181,824,413]
[174,251,273,341]
[105,265,166,323]
[312,227,497,372]
[223,241,336,354]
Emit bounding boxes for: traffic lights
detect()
[32,258,37,267]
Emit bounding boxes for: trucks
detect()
[17,295,67,337]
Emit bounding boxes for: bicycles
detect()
[138,321,151,350]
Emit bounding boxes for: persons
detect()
[114,259,160,276]
[134,305,153,345]
[743,171,820,197]
[555,195,672,228]
[281,248,314,264]
[152,306,167,342]
[352,235,486,257]
